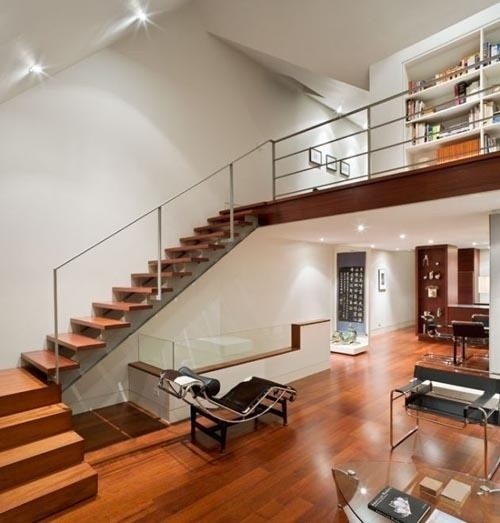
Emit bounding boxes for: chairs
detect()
[451,321,489,365]
[157,366,298,453]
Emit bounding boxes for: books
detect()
[367,483,432,522]
[443,478,472,504]
[405,40,500,164]
[419,475,443,496]
[425,507,467,523]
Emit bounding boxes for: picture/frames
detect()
[378,269,387,291]
[340,160,350,177]
[309,147,322,166]
[326,154,337,171]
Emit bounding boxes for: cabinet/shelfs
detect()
[401,18,500,171]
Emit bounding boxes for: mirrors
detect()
[458,247,490,305]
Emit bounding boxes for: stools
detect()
[390,379,500,481]
